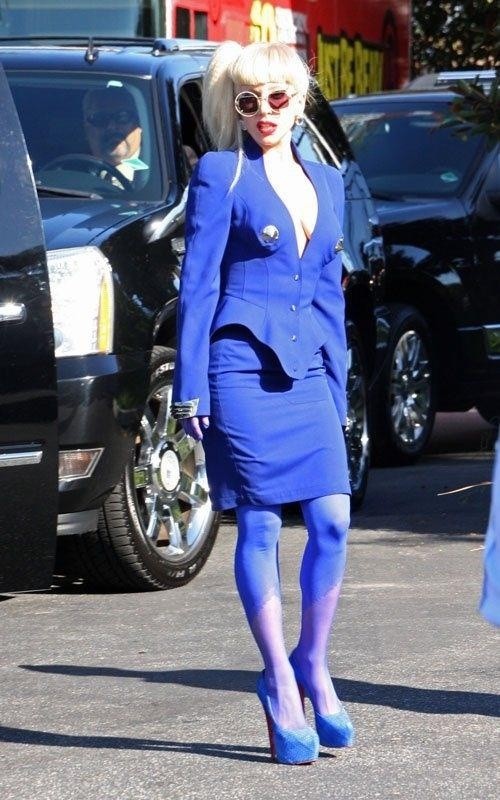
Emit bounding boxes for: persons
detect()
[83,87,149,193]
[172,43,352,763]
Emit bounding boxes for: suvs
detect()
[1,29,389,596]
[323,71,500,458]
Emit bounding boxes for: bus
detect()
[1,0,411,119]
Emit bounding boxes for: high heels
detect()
[256,669,320,764]
[289,649,353,747]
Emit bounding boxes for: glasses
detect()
[234,90,298,117]
[87,109,134,126]
